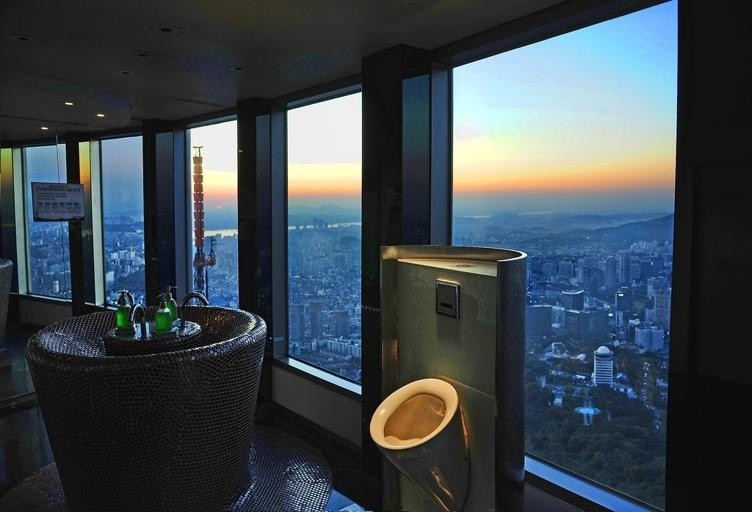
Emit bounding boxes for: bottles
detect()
[152,293,172,329]
[116,291,134,329]
[165,285,179,320]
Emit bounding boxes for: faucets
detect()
[178,292,209,330]
[129,302,147,339]
[121,288,135,307]
